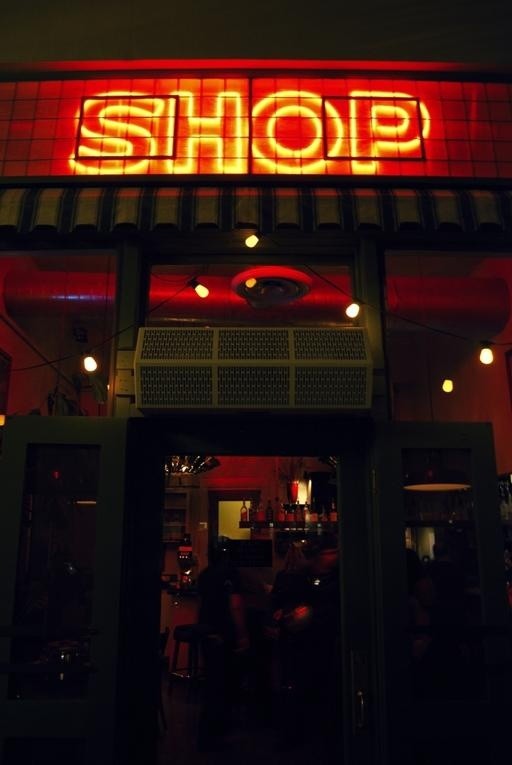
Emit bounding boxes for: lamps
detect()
[229,264,314,312]
[400,451,474,494]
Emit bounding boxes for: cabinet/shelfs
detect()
[163,485,191,546]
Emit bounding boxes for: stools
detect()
[167,625,211,697]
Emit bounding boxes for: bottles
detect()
[277,497,317,526]
[330,497,337,521]
[266,499,273,527]
[239,497,247,522]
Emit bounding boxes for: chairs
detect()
[156,628,171,735]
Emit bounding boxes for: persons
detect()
[275,531,341,724]
[196,534,255,755]
[176,531,196,581]
